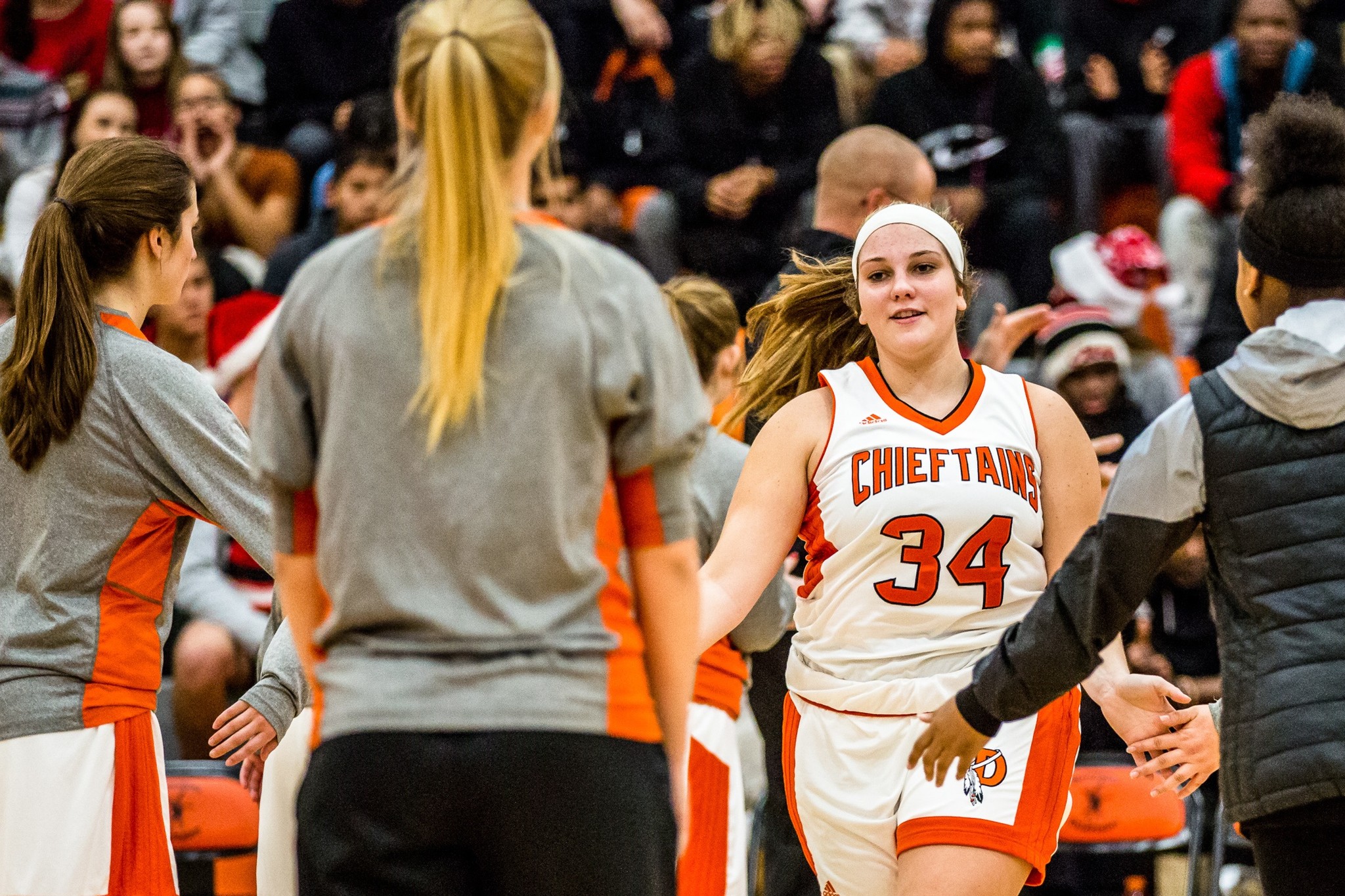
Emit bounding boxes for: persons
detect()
[246,0,713,896]
[0,134,278,896]
[906,94,1345,896]
[0,0,1345,896]
[696,199,1193,895]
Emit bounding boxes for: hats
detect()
[1098,225,1170,281]
[1030,307,1133,394]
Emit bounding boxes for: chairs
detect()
[1057,752,1206,896]
[1210,799,1254,896]
[164,757,259,896]
